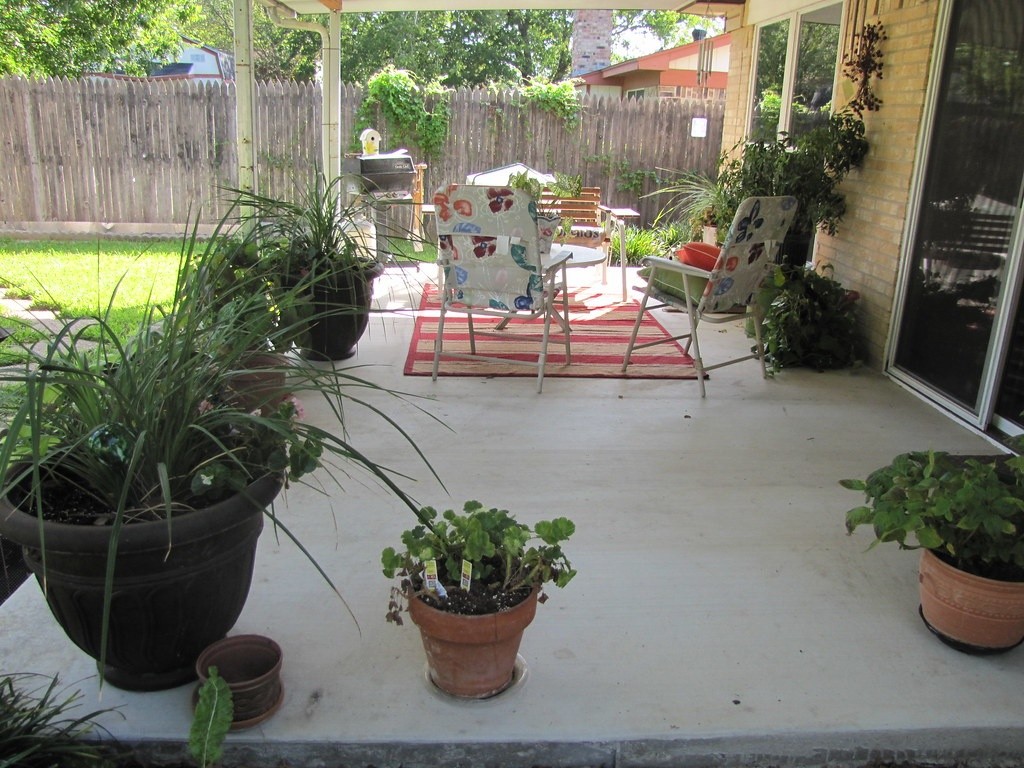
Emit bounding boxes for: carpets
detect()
[404,284,709,380]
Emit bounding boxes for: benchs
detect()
[537,187,611,284]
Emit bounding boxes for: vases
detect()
[196,634,284,720]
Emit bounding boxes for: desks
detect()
[496,243,606,331]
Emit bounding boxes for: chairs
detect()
[432,185,573,394]
[622,195,799,398]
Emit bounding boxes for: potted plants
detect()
[840,434,1024,648]
[381,500,576,699]
[715,104,868,336]
[1,158,457,692]
[507,169,582,254]
[690,207,717,246]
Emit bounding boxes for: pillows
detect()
[675,242,721,272]
[637,266,709,305]
